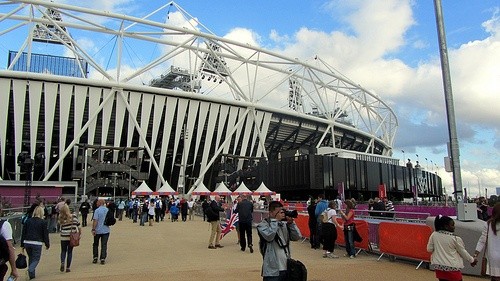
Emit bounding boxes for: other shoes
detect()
[241,247,246,251]
[214,244,224,249]
[100,259,106,264]
[60,262,65,272]
[349,254,356,259]
[93,258,98,264]
[249,245,253,253]
[323,252,339,258]
[343,251,348,257]
[66,268,70,273]
[207,245,216,249]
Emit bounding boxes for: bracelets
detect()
[336,224,339,227]
[92,229,96,231]
[474,254,477,257]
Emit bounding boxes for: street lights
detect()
[400,149,405,167]
[180,123,189,195]
[415,153,433,172]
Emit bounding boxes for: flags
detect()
[220,212,239,241]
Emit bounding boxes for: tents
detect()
[233,181,253,201]
[254,181,276,202]
[211,181,233,201]
[154,180,178,199]
[131,180,153,198]
[192,181,211,201]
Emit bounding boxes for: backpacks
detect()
[320,208,332,223]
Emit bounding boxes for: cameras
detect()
[281,209,297,221]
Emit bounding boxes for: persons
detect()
[27,197,68,233]
[201,199,209,222]
[256,200,303,281]
[79,199,91,227]
[92,196,198,226]
[426,198,500,281]
[90,199,111,265]
[20,206,50,280]
[307,196,364,260]
[368,197,395,217]
[58,205,82,272]
[0,204,19,281]
[235,192,254,254]
[207,195,225,249]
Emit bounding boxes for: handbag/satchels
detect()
[15,253,27,269]
[481,256,486,277]
[104,209,117,227]
[70,232,80,247]
[286,257,308,281]
